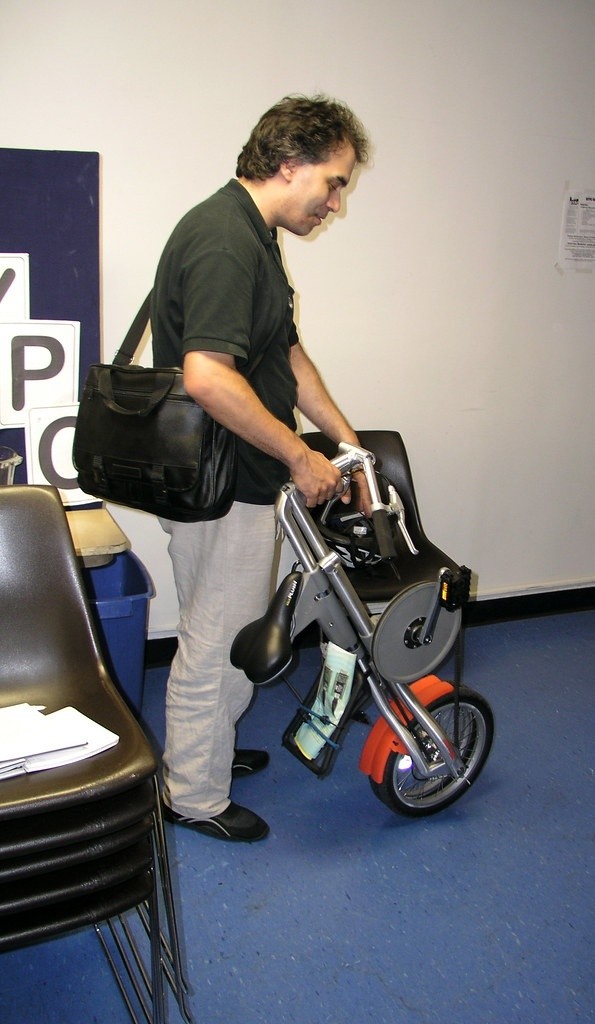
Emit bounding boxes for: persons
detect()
[147,94,378,843]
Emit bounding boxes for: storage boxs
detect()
[81,549,156,717]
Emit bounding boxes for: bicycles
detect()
[230,441,494,819]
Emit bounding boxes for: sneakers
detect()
[231,746,268,779]
[160,794,270,842]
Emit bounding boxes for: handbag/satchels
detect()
[72,361,240,524]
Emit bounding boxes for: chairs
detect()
[0,484,196,1024]
[296,430,469,681]
